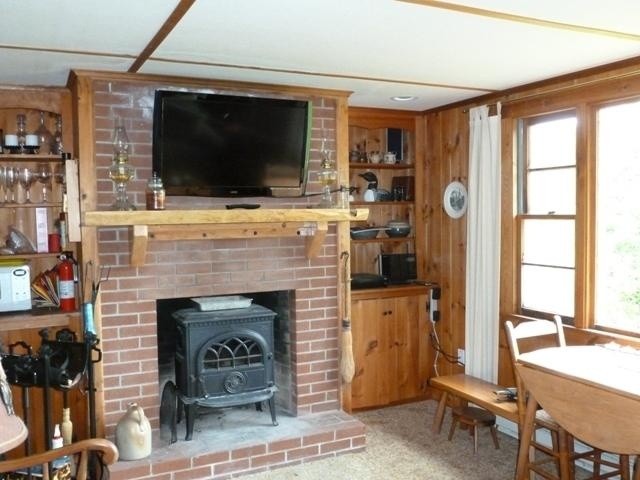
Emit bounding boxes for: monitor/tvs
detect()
[152,90,312,197]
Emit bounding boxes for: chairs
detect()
[506,314,631,480]
[0,438,118,480]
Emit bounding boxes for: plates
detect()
[351,231,381,240]
[444,182,468,218]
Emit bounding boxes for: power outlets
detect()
[457,348,465,367]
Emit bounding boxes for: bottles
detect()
[11,113,33,154]
[53,425,64,449]
[32,111,54,154]
[350,150,396,165]
[144,172,168,212]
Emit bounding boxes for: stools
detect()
[449,406,501,455]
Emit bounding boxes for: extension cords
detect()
[430,289,438,323]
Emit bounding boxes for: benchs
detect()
[427,373,576,480]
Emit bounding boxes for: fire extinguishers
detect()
[56,251,78,313]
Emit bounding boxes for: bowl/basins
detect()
[386,222,411,237]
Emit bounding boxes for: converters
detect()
[433,287,440,299]
[433,311,439,321]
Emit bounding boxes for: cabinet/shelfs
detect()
[348,107,426,287]
[0,83,80,313]
[1,316,89,461]
[348,287,439,414]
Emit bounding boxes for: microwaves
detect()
[0,265,32,313]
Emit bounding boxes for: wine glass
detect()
[1,163,52,203]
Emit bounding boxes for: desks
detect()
[515,343,639,480]
